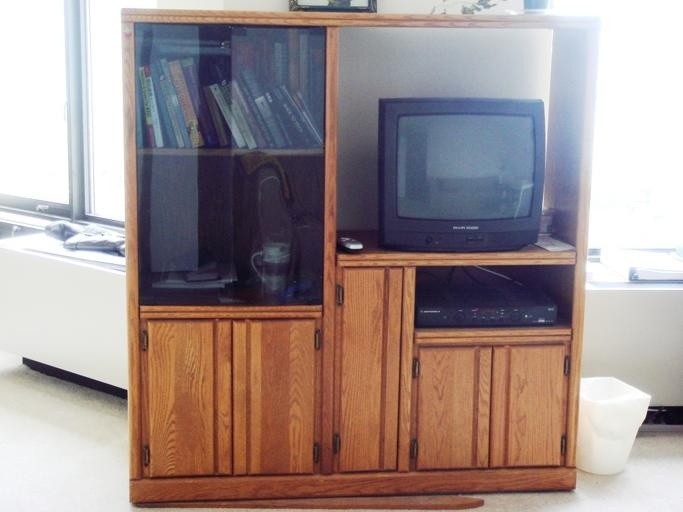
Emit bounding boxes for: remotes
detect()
[339,236,364,250]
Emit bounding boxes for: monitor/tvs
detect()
[379,98,545,252]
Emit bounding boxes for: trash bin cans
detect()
[575,376,652,474]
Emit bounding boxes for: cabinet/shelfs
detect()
[331,251,585,494]
[118,11,331,502]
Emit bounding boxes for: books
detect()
[148,242,240,293]
[138,28,327,150]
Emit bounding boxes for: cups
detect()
[252,241,294,295]
